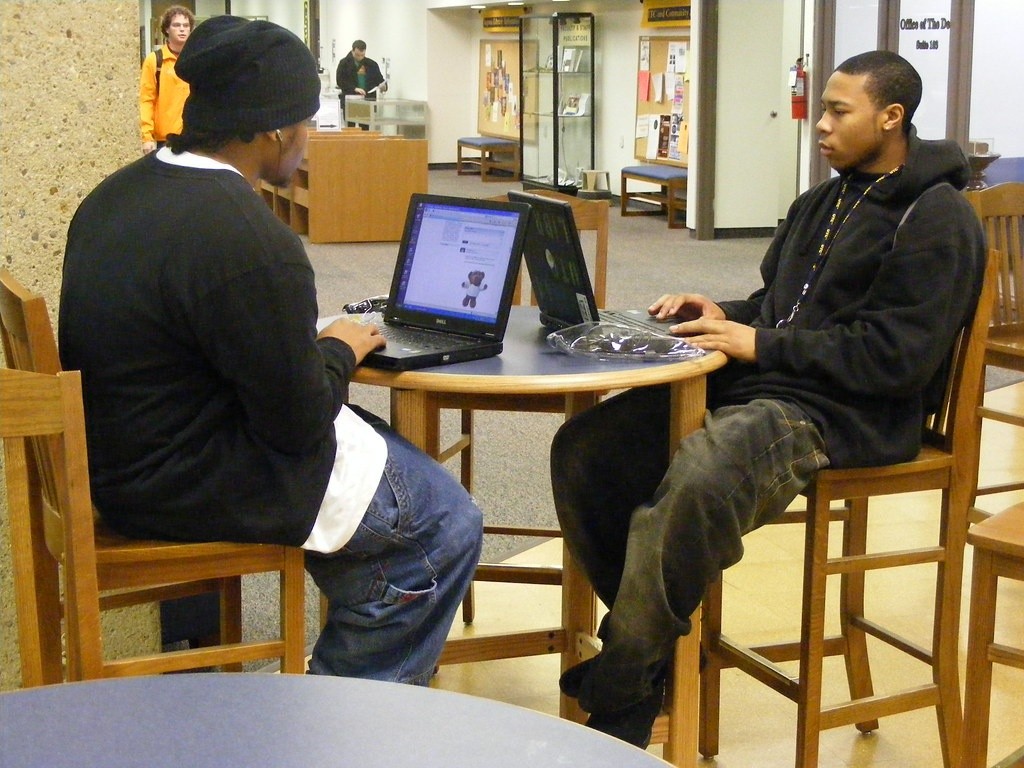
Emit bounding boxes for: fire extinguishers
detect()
[789,53,809,120]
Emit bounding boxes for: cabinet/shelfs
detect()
[253,126,431,245]
[346,97,432,138]
[517,12,595,197]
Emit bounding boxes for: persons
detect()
[58,15,484,687]
[550,50,986,751]
[138,6,195,156]
[336,39,388,131]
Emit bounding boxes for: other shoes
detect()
[560,653,601,696]
[586,716,654,749]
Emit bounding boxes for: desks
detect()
[314,305,732,768]
[0,672,682,768]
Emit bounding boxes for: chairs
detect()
[434,189,609,627]
[696,181,1024,768]
[1,267,304,689]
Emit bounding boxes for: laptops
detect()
[356,191,533,371]
[507,190,704,341]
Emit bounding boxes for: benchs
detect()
[620,163,687,230]
[456,135,522,183]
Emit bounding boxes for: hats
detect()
[175,15,321,131]
